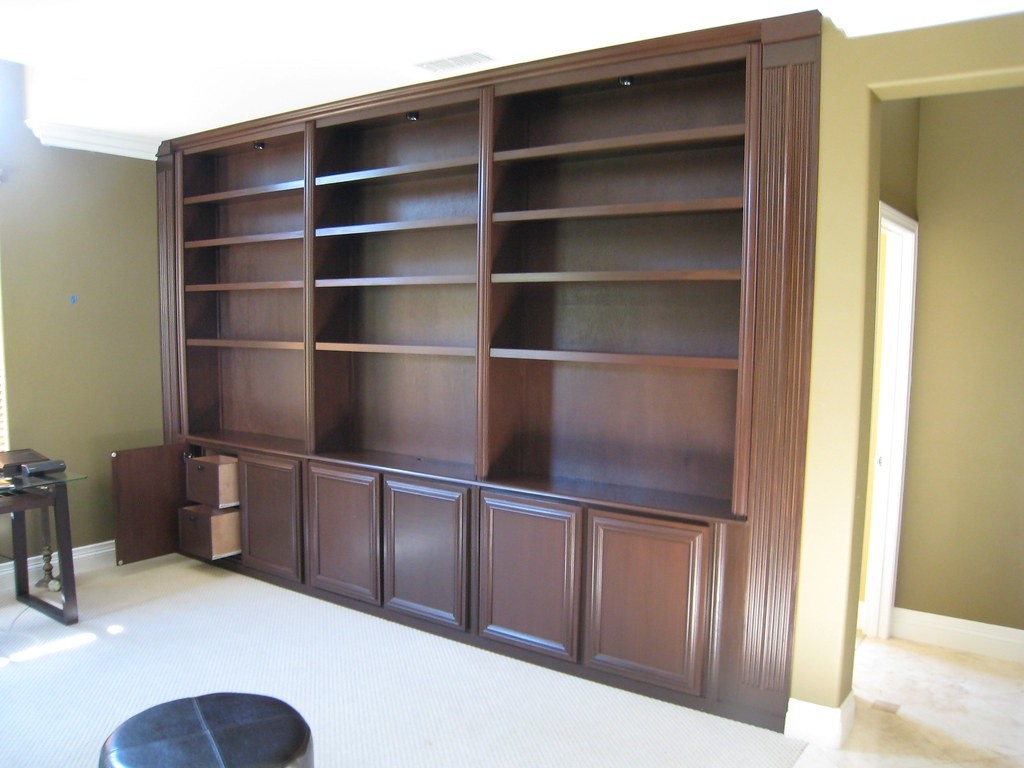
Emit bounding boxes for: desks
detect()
[0,469,90,626]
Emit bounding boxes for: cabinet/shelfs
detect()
[110,9,823,732]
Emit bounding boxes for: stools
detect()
[99,692,315,768]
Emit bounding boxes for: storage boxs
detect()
[173,452,242,563]
